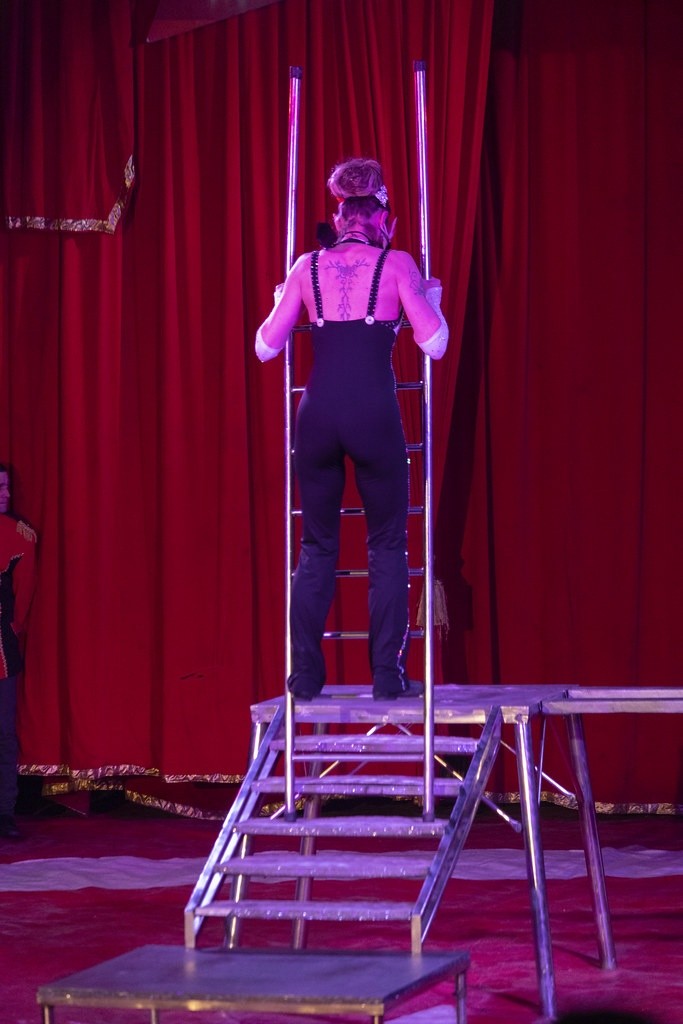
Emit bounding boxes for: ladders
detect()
[284,60,435,823]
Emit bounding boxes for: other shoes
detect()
[293,682,315,701]
[0,816,20,839]
[371,680,426,701]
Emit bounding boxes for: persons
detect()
[0,461,37,841]
[255,159,449,702]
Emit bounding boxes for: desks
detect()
[36,944,470,1024]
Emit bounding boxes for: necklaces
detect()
[340,231,372,242]
